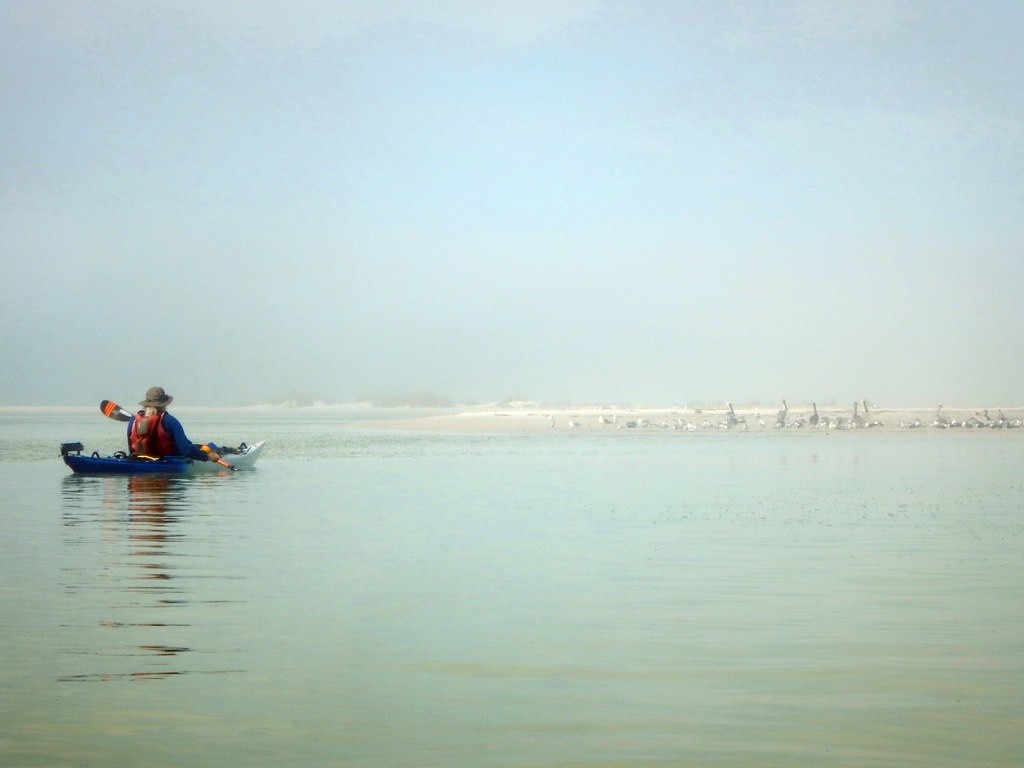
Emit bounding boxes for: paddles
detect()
[99,399,243,472]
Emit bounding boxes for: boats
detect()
[60,440,268,476]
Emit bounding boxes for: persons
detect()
[127,387,247,462]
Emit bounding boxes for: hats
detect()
[139,387,173,407]
